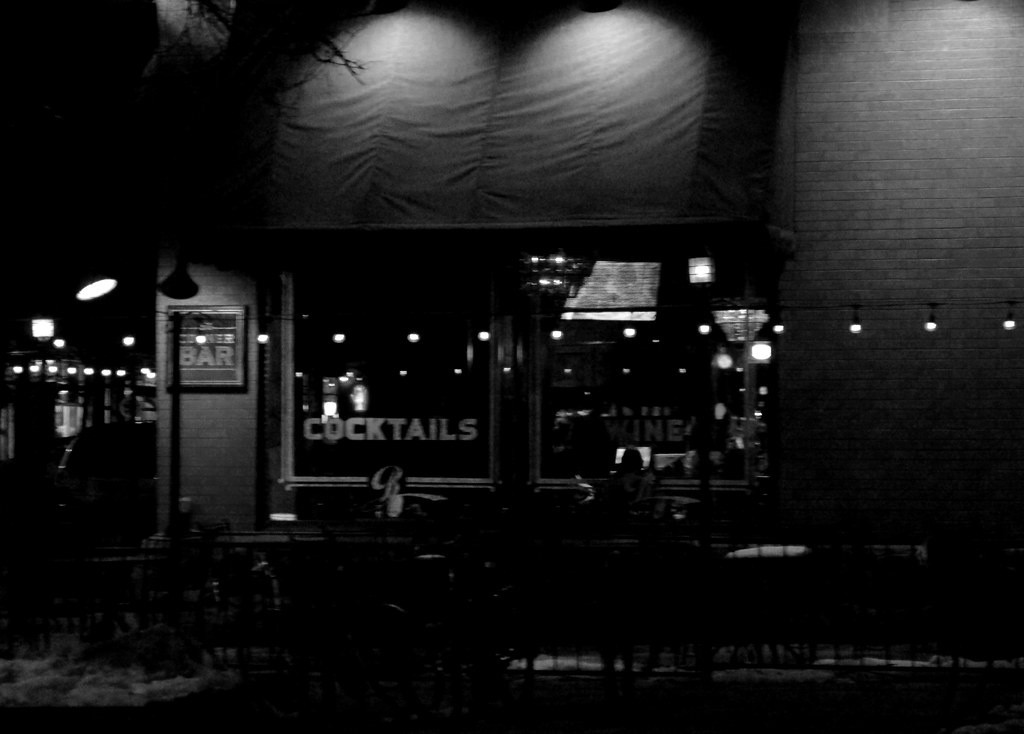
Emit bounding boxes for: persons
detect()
[619,448,643,473]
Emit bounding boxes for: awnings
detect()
[256,0,767,229]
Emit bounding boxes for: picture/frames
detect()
[165,303,248,390]
